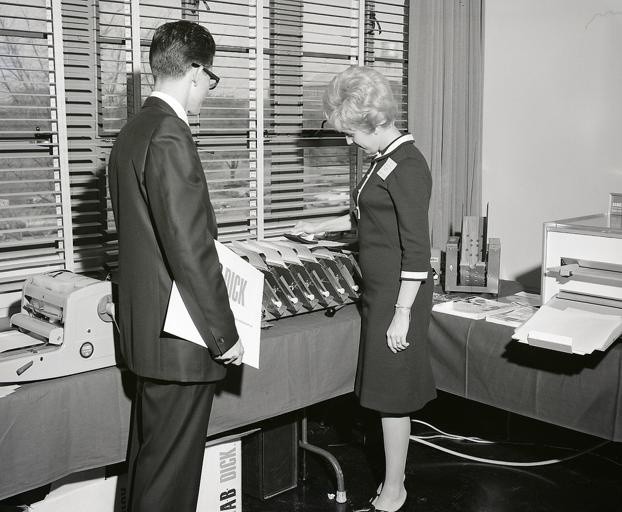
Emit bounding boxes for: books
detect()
[433,288,543,329]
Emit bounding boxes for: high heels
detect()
[351,473,409,512]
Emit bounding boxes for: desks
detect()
[0,279,622,512]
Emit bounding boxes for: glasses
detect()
[192,63,219,90]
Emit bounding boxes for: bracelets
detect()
[395,303,412,313]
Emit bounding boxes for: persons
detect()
[320,66,439,512]
[106,20,244,512]
